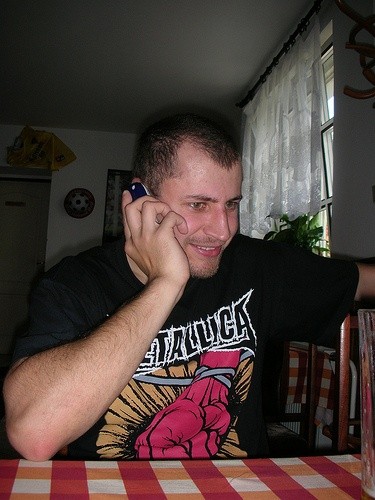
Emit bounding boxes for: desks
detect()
[0,453,375,500]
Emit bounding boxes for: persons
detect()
[2,113,375,462]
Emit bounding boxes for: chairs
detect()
[262,304,361,453]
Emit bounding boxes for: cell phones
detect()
[126,182,150,201]
